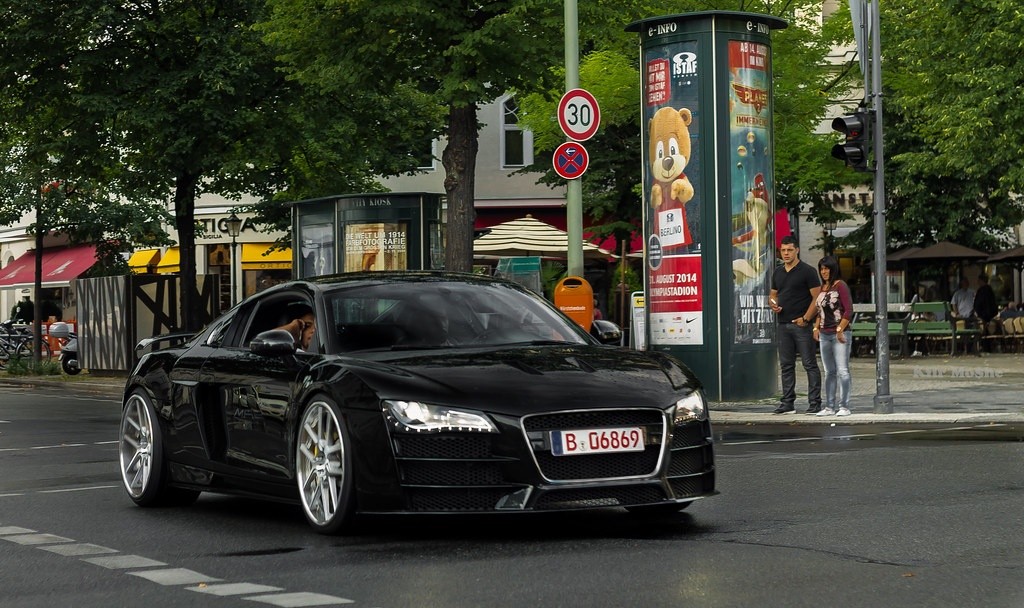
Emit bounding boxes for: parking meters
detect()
[554,278,593,355]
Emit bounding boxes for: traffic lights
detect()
[830,112,869,171]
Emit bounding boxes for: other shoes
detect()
[805,405,821,414]
[773,403,796,415]
[816,408,837,415]
[836,409,851,416]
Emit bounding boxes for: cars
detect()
[119,270,720,534]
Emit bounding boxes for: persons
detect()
[271,306,316,352]
[593,300,602,320]
[1000,301,1018,319]
[812,257,853,416]
[768,236,821,414]
[973,274,998,321]
[950,277,977,326]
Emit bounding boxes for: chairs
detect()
[913,317,1024,355]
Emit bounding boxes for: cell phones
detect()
[770,299,777,306]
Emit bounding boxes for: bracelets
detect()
[836,327,842,333]
[802,317,809,323]
[813,328,817,331]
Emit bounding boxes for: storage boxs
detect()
[49,322,69,338]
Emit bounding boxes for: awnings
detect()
[242,244,292,270]
[157,248,181,273]
[13,239,120,288]
[127,250,160,273]
[0,245,67,290]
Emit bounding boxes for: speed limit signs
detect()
[557,88,601,142]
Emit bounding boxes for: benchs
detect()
[849,301,985,359]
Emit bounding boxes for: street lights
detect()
[226,207,242,308]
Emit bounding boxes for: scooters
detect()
[49,322,82,375]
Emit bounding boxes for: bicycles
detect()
[0,307,51,371]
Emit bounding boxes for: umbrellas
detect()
[903,239,991,300]
[979,245,1024,303]
[867,247,924,300]
[473,215,611,259]
